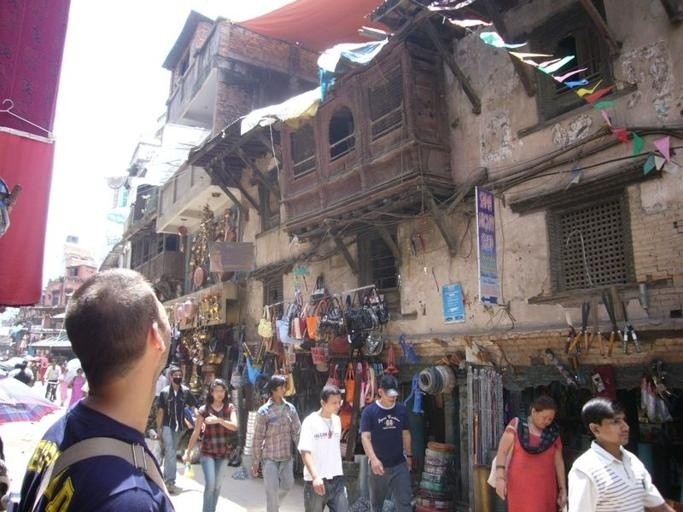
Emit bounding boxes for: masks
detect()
[173,378,182,384]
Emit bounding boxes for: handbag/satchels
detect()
[145,437,163,467]
[257,288,390,364]
[323,377,378,456]
[225,432,241,466]
[487,453,511,488]
[256,365,297,397]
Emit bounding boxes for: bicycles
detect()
[45,379,60,403]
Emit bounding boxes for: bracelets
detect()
[497,477,506,481]
[496,465,505,470]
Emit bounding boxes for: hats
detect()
[381,376,399,396]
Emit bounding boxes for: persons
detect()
[496,396,567,511]
[13,360,238,511]
[18,268,176,512]
[252,375,414,512]
[565,399,683,512]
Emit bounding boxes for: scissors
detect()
[564,310,580,356]
[568,302,589,354]
[587,297,605,355]
[622,302,640,354]
[602,291,632,357]
[639,284,663,324]
